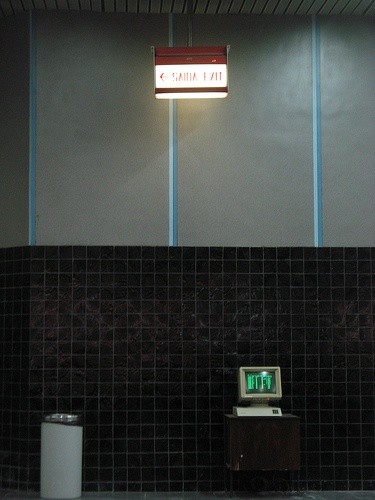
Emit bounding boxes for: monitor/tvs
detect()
[239,366,283,405]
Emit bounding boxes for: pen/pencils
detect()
[38,413,83,499]
